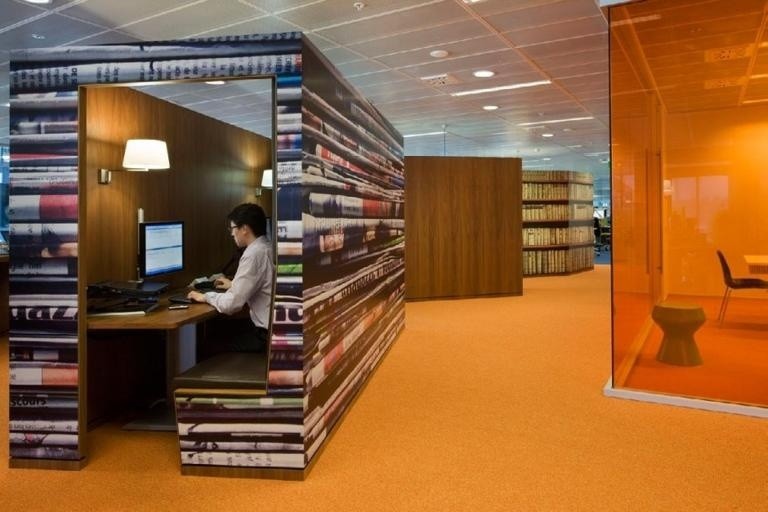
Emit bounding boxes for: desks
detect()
[742,255,768,274]
[86,286,219,431]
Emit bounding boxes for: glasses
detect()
[227,225,240,231]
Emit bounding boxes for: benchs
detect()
[172,349,266,396]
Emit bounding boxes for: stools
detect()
[652,303,707,366]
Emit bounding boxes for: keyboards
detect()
[168,288,217,304]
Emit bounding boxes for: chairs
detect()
[716,250,768,329]
[593,217,611,255]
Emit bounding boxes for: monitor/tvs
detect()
[140,221,185,278]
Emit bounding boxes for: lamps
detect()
[98,138,171,185]
[255,168,274,198]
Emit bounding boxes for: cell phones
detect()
[168,306,189,310]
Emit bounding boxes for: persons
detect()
[187,202,275,363]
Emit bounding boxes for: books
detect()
[521,167,598,274]
